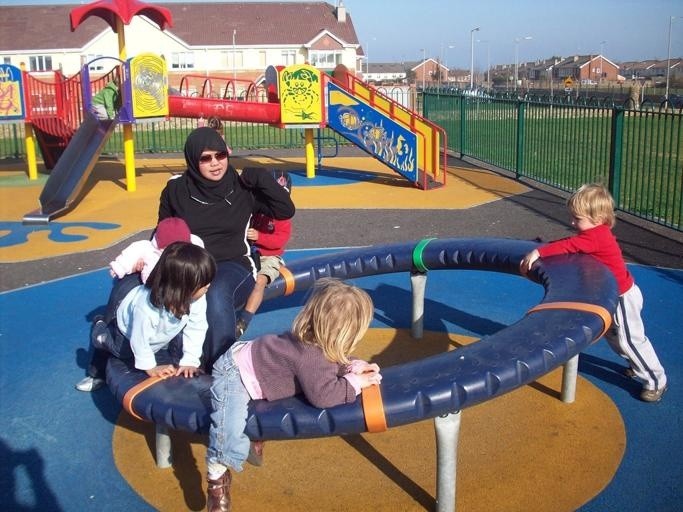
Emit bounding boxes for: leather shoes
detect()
[205,468,232,512]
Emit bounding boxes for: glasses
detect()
[198,151,226,164]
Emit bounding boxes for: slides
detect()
[22,112,120,225]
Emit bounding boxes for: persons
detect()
[75,121,296,391]
[90,73,120,120]
[517,181,670,403]
[234,169,292,339]
[203,275,383,512]
[110,217,207,286]
[90,241,215,379]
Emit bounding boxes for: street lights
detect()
[419,24,533,96]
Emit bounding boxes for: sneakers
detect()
[92,314,105,333]
[639,383,668,402]
[75,375,106,392]
[235,315,247,340]
[624,367,637,377]
